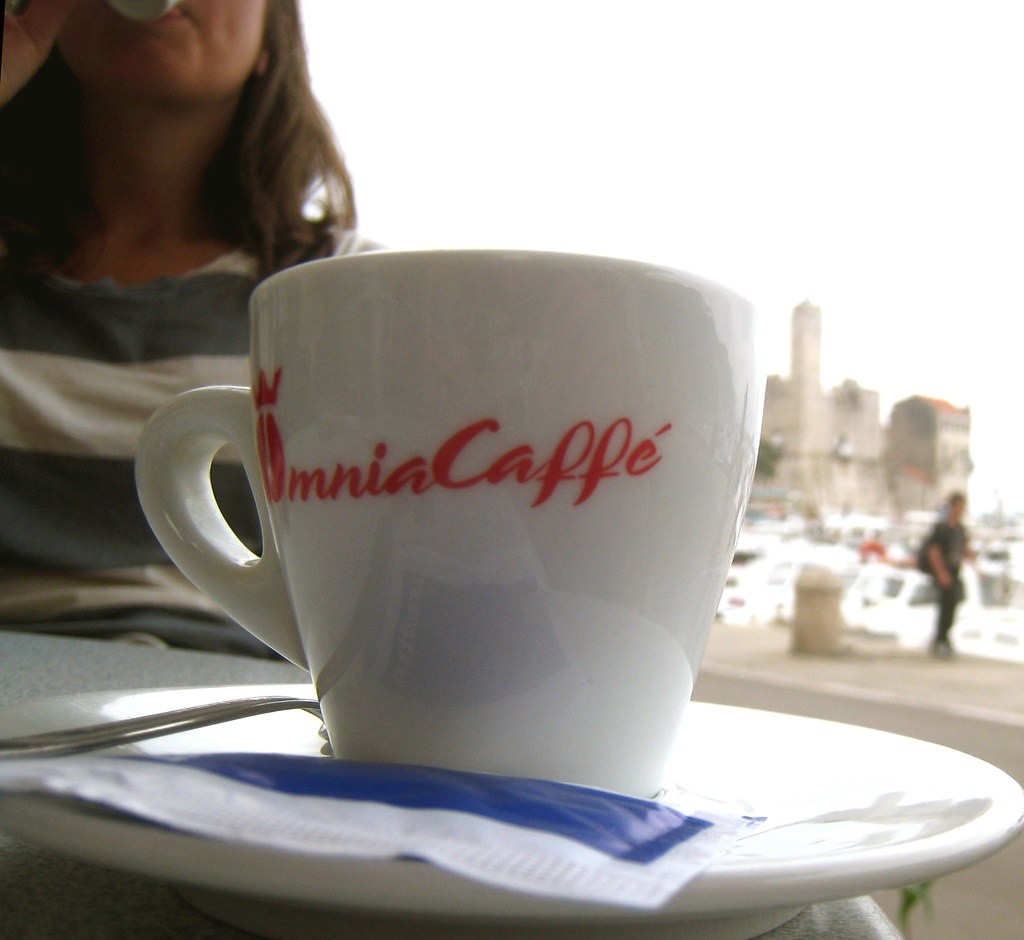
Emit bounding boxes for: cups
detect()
[136,247,768,796]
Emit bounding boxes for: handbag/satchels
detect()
[916,522,945,575]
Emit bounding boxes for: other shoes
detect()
[928,637,953,657]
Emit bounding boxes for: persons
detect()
[919,492,970,659]
[0,0,396,655]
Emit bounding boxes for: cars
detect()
[711,511,1024,664]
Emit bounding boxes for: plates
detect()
[0,681,1024,940]
